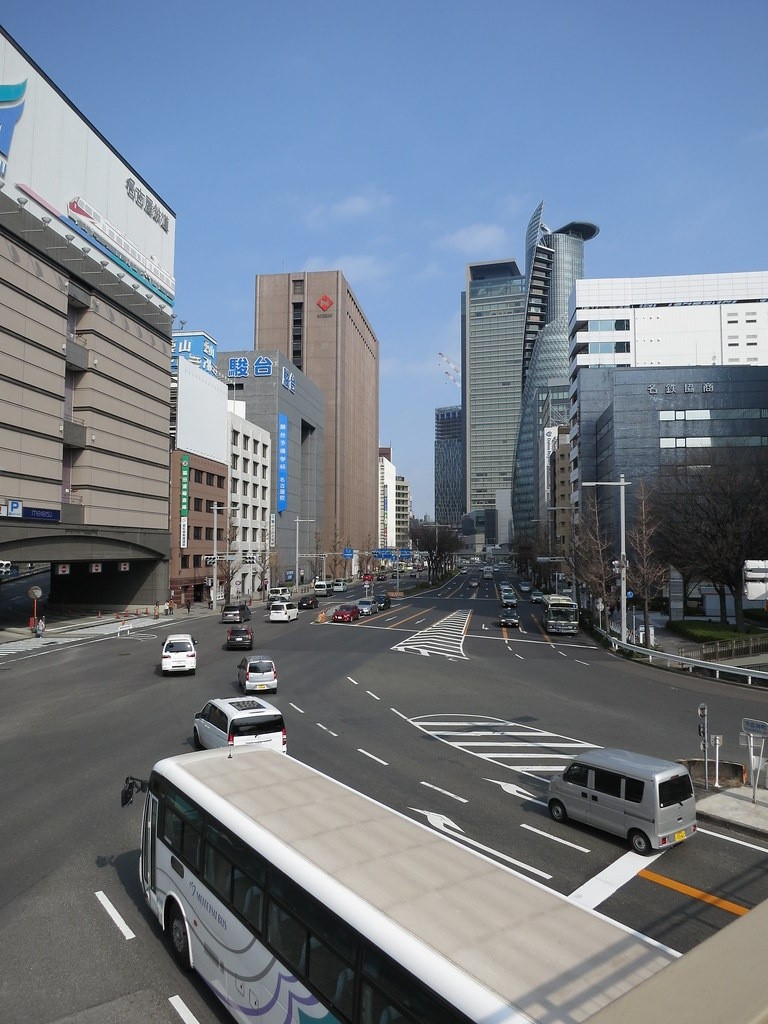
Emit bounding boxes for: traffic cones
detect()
[144,607,149,614]
[134,609,140,615]
[97,611,102,618]
[124,610,129,617]
[116,612,121,619]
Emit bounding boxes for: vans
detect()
[161,633,199,675]
[313,581,334,597]
[192,695,288,756]
[237,655,278,695]
[266,587,300,623]
[547,748,698,857]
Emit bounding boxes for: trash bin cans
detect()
[208,601,214,610]
[639,624,655,646]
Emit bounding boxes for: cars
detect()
[373,593,391,610]
[357,598,379,616]
[362,560,518,608]
[331,604,361,622]
[531,591,543,603]
[499,607,521,628]
[333,581,347,591]
[226,624,255,650]
[298,595,319,608]
[221,603,253,623]
[518,581,531,592]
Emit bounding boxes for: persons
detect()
[36,619,45,638]
[207,598,211,609]
[186,600,192,614]
[153,604,159,620]
[164,599,175,616]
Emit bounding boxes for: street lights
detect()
[581,473,632,647]
[293,516,315,593]
[210,501,240,612]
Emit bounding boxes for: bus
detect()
[119,740,688,1024]
[541,594,579,635]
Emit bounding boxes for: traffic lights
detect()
[0,560,11,576]
